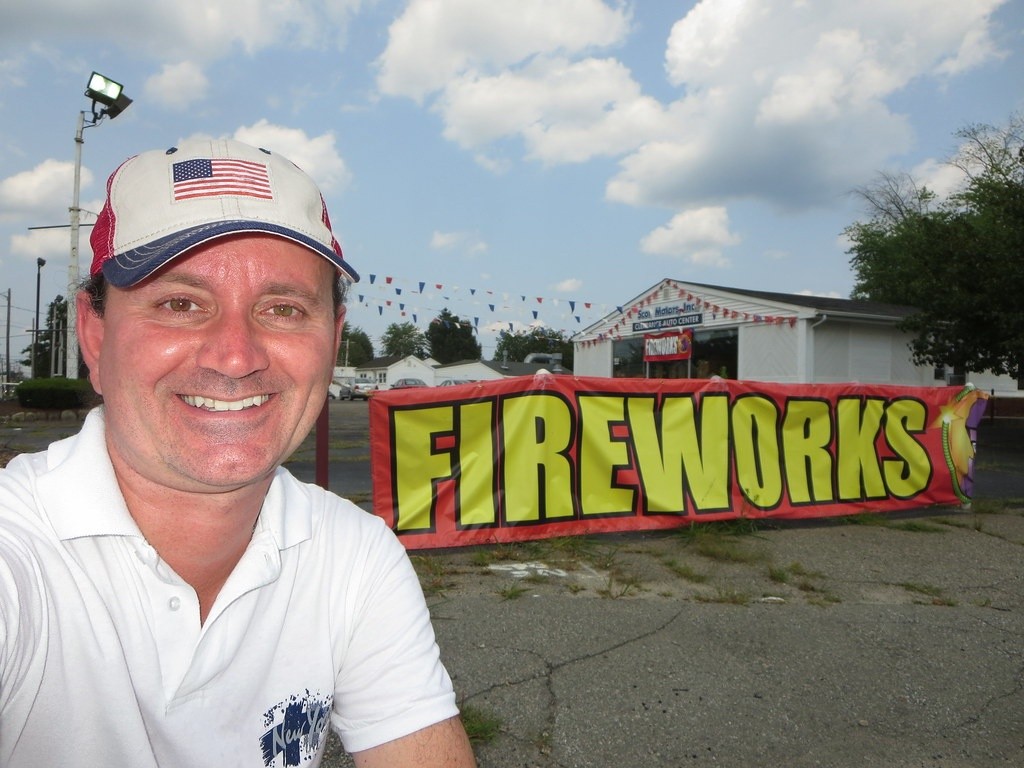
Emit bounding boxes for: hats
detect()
[91,136,360,287]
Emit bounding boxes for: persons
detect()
[0,139,475,768]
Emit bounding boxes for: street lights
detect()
[51,295,63,378]
[65,70,134,380]
[34,258,46,377]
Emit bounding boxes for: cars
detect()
[329,375,377,401]
[436,380,471,387]
[390,377,428,390]
